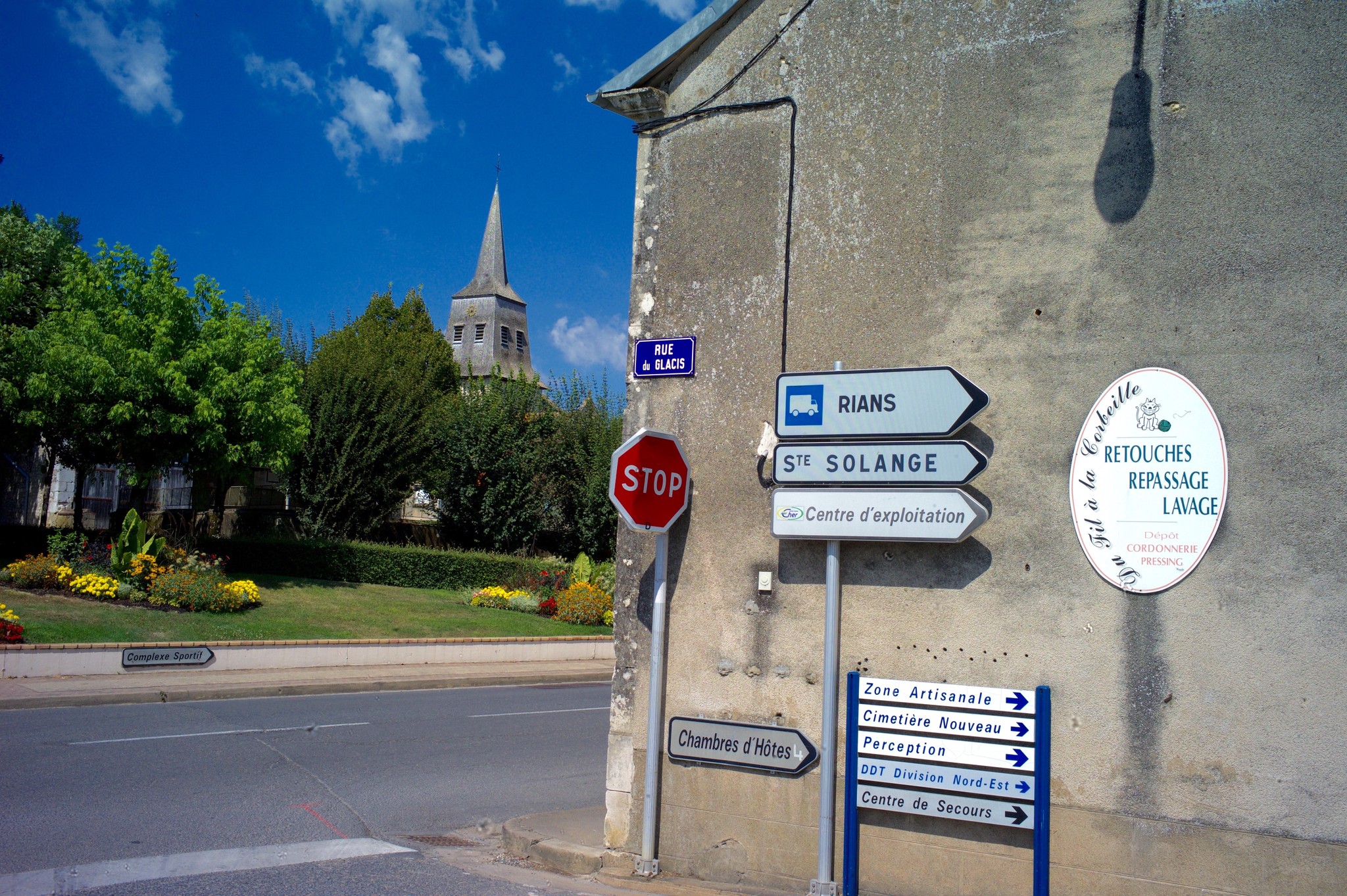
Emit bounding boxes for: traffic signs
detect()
[775,440,988,483]
[775,366,990,439]
[770,489,988,543]
[857,677,1034,829]
[667,716,818,775]
[123,646,215,666]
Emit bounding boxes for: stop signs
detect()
[608,428,691,533]
